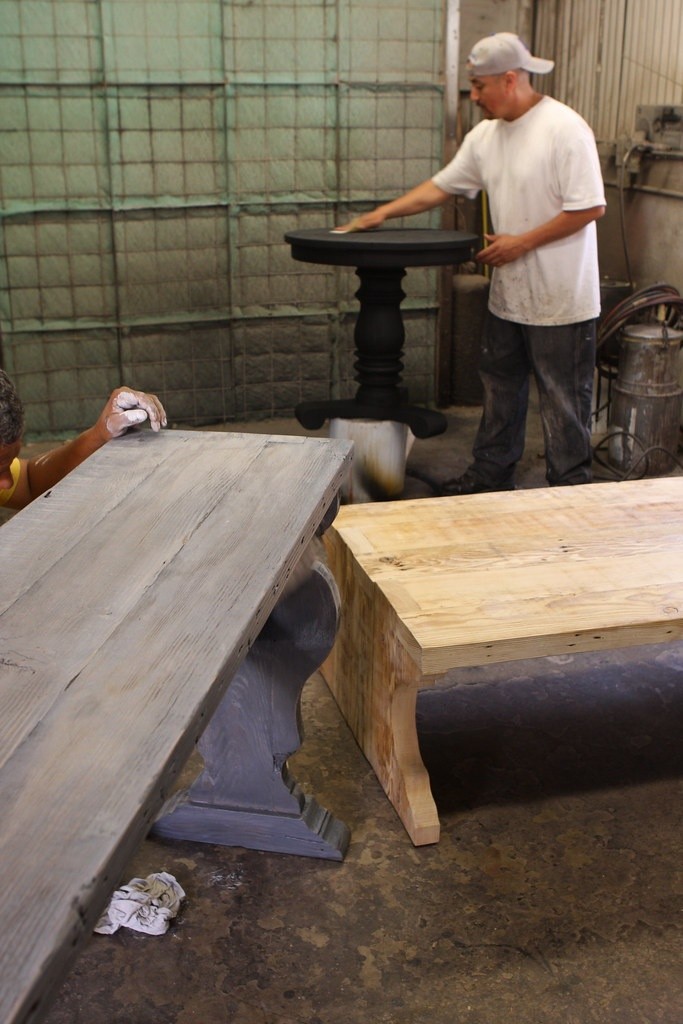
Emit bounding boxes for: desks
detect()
[311,474,680,846]
[282,226,477,438]
[0,424,359,1022]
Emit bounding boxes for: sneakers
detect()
[438,468,490,495]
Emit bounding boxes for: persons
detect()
[342,31,611,500]
[0,369,172,527]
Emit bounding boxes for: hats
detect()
[463,31,554,78]
